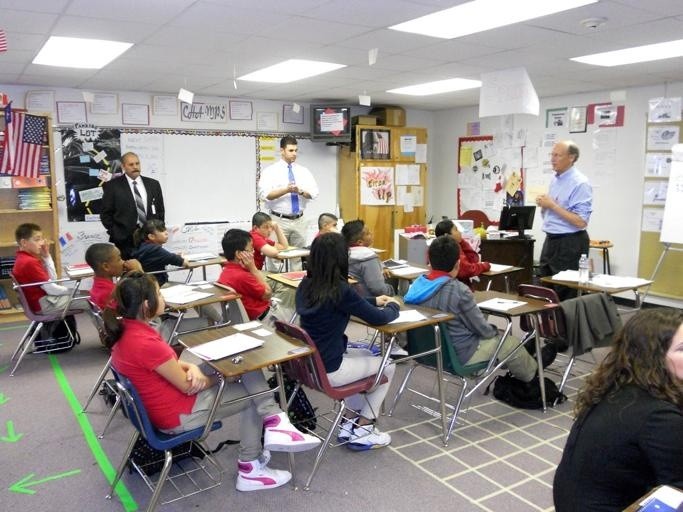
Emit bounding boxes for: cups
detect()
[426,224,436,236]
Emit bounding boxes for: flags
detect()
[0,106,47,182]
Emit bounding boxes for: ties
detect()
[288,165,299,213]
[132,181,147,228]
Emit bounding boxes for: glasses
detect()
[244,248,254,252]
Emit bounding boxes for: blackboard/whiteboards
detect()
[52,127,341,296]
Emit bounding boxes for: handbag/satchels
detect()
[485,372,568,409]
[127,439,241,477]
[22,315,80,355]
[267,374,317,433]
[100,378,121,407]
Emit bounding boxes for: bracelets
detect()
[213,369,223,385]
[298,189,304,196]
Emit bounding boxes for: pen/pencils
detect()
[497,302,517,304]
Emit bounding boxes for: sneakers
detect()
[536,344,557,375]
[337,415,380,442]
[264,412,321,453]
[236,451,293,492]
[347,419,391,451]
[522,334,535,356]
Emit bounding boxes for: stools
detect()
[588,239,611,274]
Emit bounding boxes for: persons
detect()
[534,138,590,372]
[550,304,683,511]
[403,236,556,382]
[108,268,325,496]
[84,241,216,346]
[218,225,300,332]
[257,133,320,271]
[130,217,226,326]
[247,211,289,268]
[424,219,491,286]
[294,230,401,452]
[311,212,344,243]
[98,152,166,262]
[342,219,408,297]
[11,221,106,346]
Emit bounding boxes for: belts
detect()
[258,307,270,320]
[546,229,586,239]
[271,210,303,219]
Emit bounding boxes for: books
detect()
[1,131,54,315]
[280,271,307,280]
[66,262,91,270]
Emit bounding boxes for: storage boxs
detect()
[367,105,405,126]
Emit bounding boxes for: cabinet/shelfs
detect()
[0,105,61,323]
[336,127,428,262]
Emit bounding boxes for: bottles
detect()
[478,221,486,241]
[578,253,591,286]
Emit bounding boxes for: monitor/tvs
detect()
[310,104,352,143]
[499,206,536,239]
